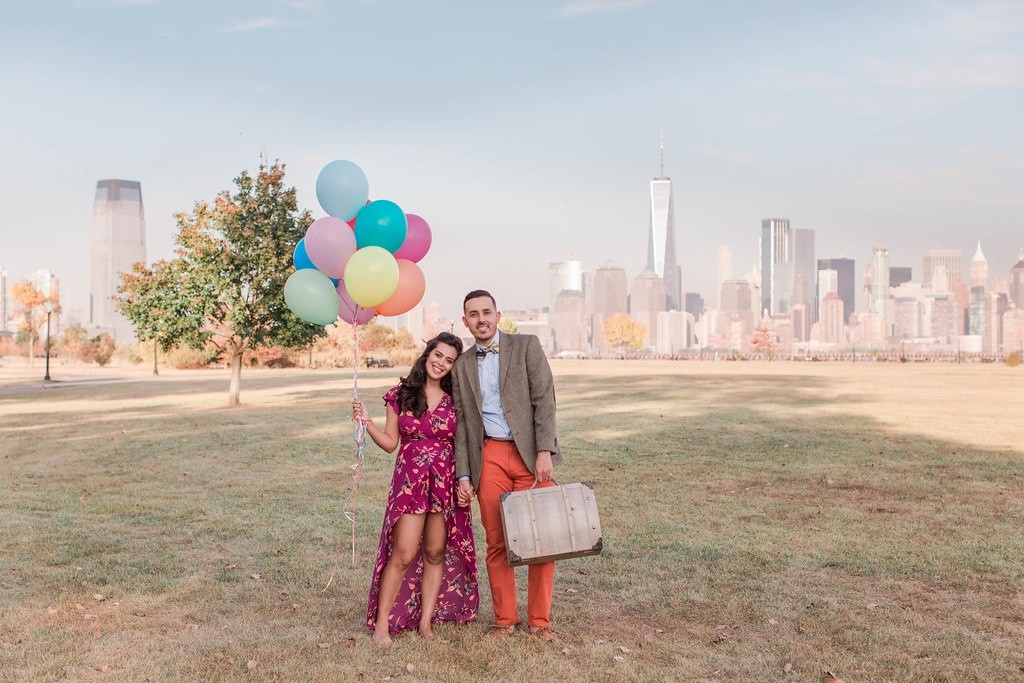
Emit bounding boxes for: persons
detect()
[353,290,564,646]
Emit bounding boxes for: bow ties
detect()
[477,342,500,359]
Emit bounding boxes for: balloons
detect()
[282,159,433,328]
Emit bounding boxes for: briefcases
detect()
[501,481,601,566]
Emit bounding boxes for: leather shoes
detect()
[529,626,560,642]
[484,626,515,640]
